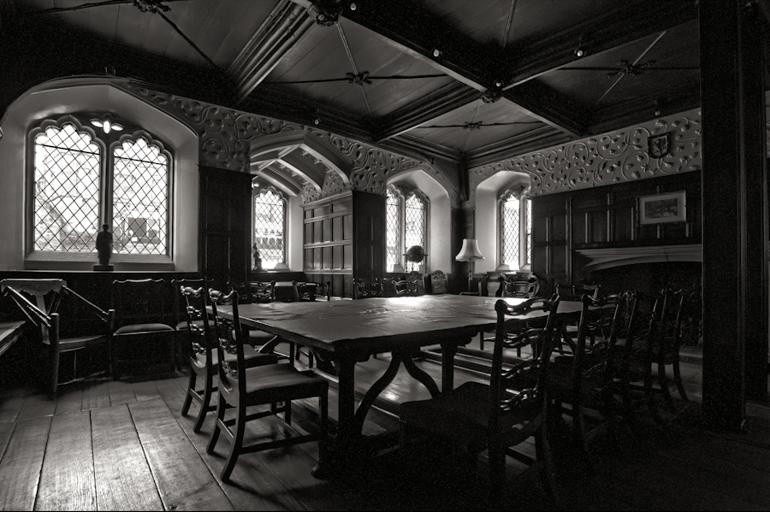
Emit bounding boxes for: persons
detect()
[96,224,113,265]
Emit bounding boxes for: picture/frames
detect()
[636,188,690,228]
[646,132,672,160]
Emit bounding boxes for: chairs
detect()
[395,286,686,504]
[207,289,328,482]
[350,277,530,301]
[0,277,115,397]
[116,276,224,378]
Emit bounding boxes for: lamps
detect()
[454,237,484,294]
